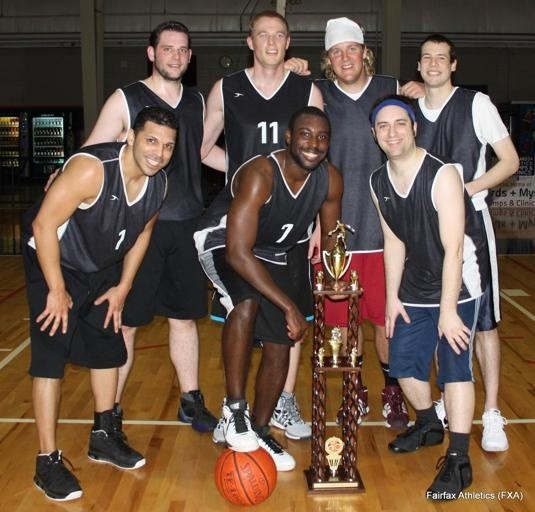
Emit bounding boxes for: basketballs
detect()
[215,447,276,505]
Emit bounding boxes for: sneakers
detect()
[426,447,472,502]
[253,425,296,473]
[481,409,509,453]
[87,404,147,470]
[336,386,371,427]
[178,390,219,433]
[381,386,411,432]
[269,391,313,441]
[211,396,260,452]
[32,448,83,502]
[388,398,450,453]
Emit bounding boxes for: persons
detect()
[201,11,325,443]
[44,21,225,446]
[282,17,426,431]
[188,107,351,473]
[407,35,521,452]
[21,106,180,502]
[369,95,490,502]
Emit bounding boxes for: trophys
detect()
[303,219,367,493]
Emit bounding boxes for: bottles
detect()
[0,117,20,167]
[517,156,535,176]
[34,119,65,185]
